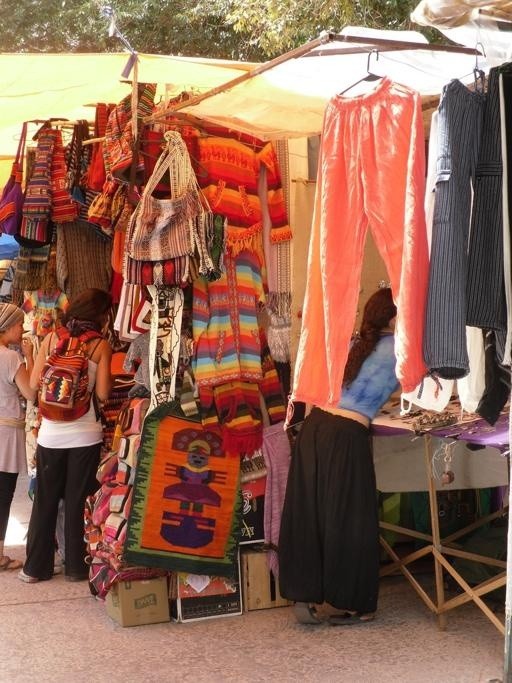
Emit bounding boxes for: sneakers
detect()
[64,576,82,582]
[18,568,39,583]
[0,555,23,572]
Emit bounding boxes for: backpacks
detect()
[37,326,102,421]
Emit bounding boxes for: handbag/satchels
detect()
[0,121,200,291]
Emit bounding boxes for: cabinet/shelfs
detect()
[369,395,510,638]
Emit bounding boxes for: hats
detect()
[0,303,23,332]
[67,289,112,320]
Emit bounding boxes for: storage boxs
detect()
[102,546,299,627]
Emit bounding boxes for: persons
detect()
[277,285,405,627]
[1,301,40,573]
[13,286,116,583]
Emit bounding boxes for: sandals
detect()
[327,611,369,625]
[294,602,321,624]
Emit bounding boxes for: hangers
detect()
[338,47,386,100]
[130,140,208,178]
[455,40,489,94]
